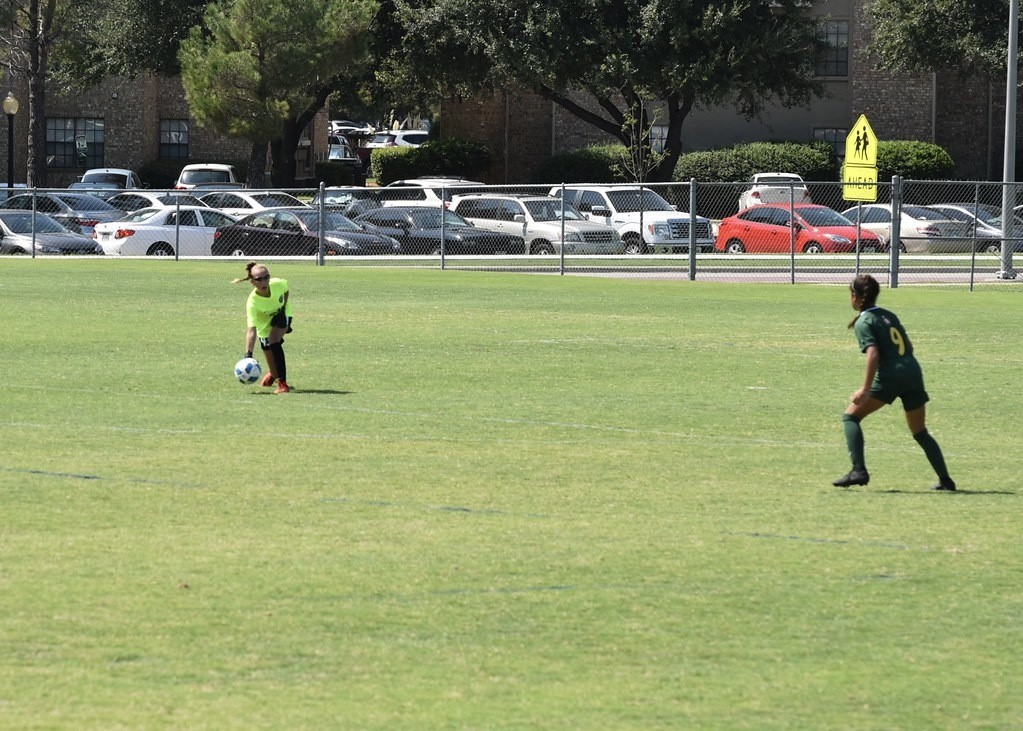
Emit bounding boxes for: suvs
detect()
[363,130,430,150]
[544,184,717,256]
[375,176,485,208]
[447,192,626,256]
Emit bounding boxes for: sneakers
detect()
[274,382,288,395]
[833,468,868,487]
[262,371,275,386]
[932,480,955,491]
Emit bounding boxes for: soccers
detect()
[234,357,262,385]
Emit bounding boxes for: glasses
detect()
[252,274,270,282]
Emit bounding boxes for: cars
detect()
[105,189,208,220]
[739,172,811,212]
[0,193,130,240]
[350,205,525,255]
[0,183,28,203]
[191,183,245,199]
[93,205,239,257]
[326,120,369,162]
[715,201,882,255]
[82,167,150,191]
[923,200,1023,254]
[198,189,312,224]
[0,208,106,257]
[305,187,383,218]
[840,203,976,253]
[68,181,119,201]
[174,162,247,189]
[211,208,400,257]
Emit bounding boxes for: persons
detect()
[231,262,295,395]
[831,275,956,494]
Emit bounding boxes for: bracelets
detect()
[244,352,252,358]
